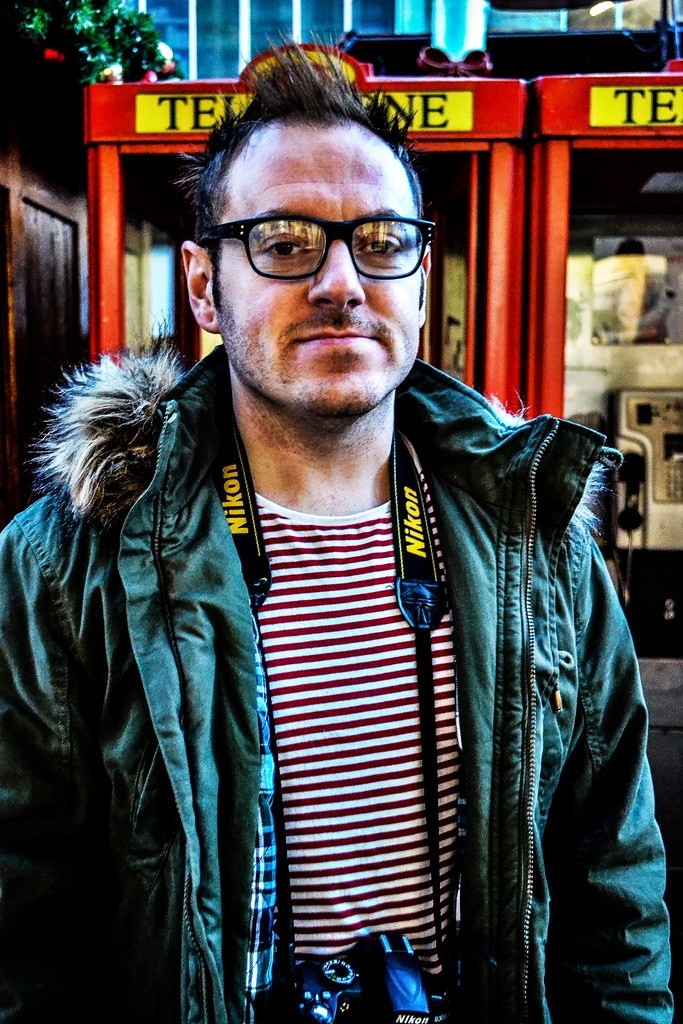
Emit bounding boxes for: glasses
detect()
[195,213,436,280]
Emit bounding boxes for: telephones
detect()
[605,390,683,551]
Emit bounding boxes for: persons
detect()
[2,33,674,1023]
[598,236,670,347]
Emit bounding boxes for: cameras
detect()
[293,932,450,1024]
